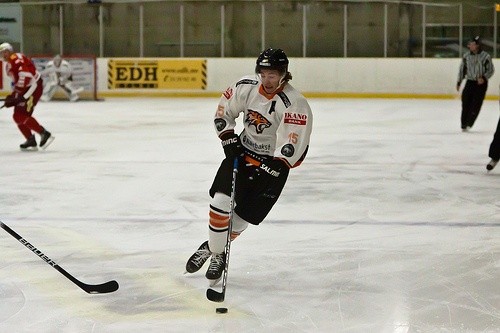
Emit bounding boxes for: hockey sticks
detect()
[0,222,119,294]
[0,103,7,109]
[205,158,238,303]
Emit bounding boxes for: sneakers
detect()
[184,240,226,288]
[39,130,55,150]
[485,158,500,172]
[19,134,38,151]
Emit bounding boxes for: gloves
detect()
[255,158,283,185]
[219,129,245,160]
[5,92,29,107]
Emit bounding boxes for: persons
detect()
[40,54,80,102]
[457,34,494,132]
[486,118,500,173]
[185,48,313,287]
[0,43,55,151]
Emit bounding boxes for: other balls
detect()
[216,307,227,313]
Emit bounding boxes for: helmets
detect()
[255,48,289,92]
[467,33,480,45]
[0,43,13,61]
[54,54,61,67]
